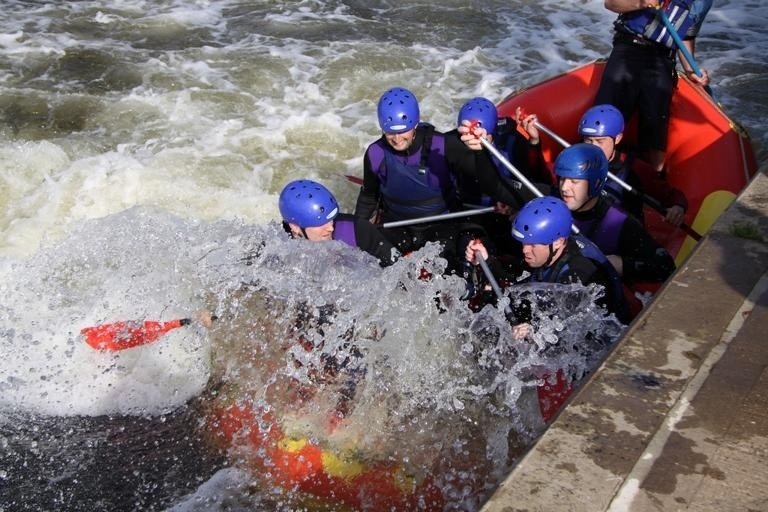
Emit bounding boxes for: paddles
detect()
[474,239,574,422]
[343,174,489,209]
[81,316,216,352]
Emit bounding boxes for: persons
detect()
[354,87,503,249]
[457,121,676,285]
[466,198,633,349]
[200,180,403,344]
[594,0,714,180]
[516,103,691,228]
[459,98,556,195]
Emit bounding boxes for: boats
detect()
[205,56,758,511]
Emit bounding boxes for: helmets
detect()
[279,181,339,226]
[378,87,419,134]
[553,143,607,198]
[512,195,574,245]
[458,98,497,138]
[576,104,625,138]
